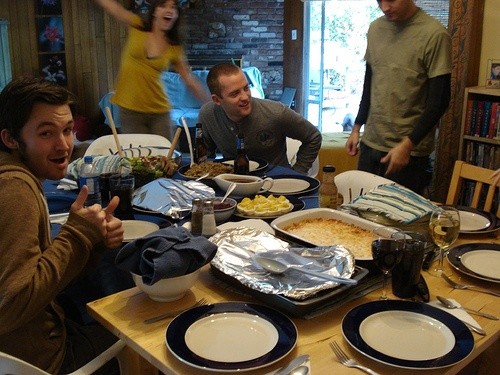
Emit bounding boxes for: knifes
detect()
[272,354,310,375]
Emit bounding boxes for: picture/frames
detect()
[486,58,500,88]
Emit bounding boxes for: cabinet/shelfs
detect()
[459,86,500,209]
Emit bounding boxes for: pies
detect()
[283,218,383,259]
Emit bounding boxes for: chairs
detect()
[447,159,500,217]
[286,136,319,178]
[334,169,396,204]
[0,339,127,375]
[84,134,174,158]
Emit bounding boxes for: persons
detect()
[198,62,322,175]
[98,0,212,151]
[345,0,453,197]
[0,76,125,375]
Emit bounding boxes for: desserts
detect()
[237,195,294,217]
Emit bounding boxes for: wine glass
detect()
[370,226,405,300]
[428,206,460,277]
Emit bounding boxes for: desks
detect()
[319,129,364,185]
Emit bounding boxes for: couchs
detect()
[98,66,266,151]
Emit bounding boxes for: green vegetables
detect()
[124,156,163,177]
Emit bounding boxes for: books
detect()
[461,96,500,216]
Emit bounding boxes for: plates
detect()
[445,242,500,282]
[216,156,320,218]
[438,204,500,234]
[210,262,369,317]
[118,215,173,244]
[341,299,475,370]
[164,301,298,373]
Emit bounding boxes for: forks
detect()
[143,298,208,324]
[440,273,500,297]
[328,340,381,375]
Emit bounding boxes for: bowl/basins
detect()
[114,146,182,178]
[212,197,237,221]
[178,162,235,187]
[269,207,412,269]
[127,267,198,306]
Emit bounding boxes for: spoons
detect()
[256,257,358,286]
[288,366,308,375]
[435,295,499,321]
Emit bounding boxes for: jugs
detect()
[212,173,274,199]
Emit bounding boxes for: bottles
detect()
[78,156,101,208]
[193,122,208,163]
[318,165,338,209]
[189,198,216,237]
[233,133,249,175]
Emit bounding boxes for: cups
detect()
[99,173,134,219]
[390,231,425,298]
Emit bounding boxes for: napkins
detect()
[47,213,69,226]
[57,181,78,189]
[426,299,486,335]
[266,357,312,375]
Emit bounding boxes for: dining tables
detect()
[42,158,500,375]
